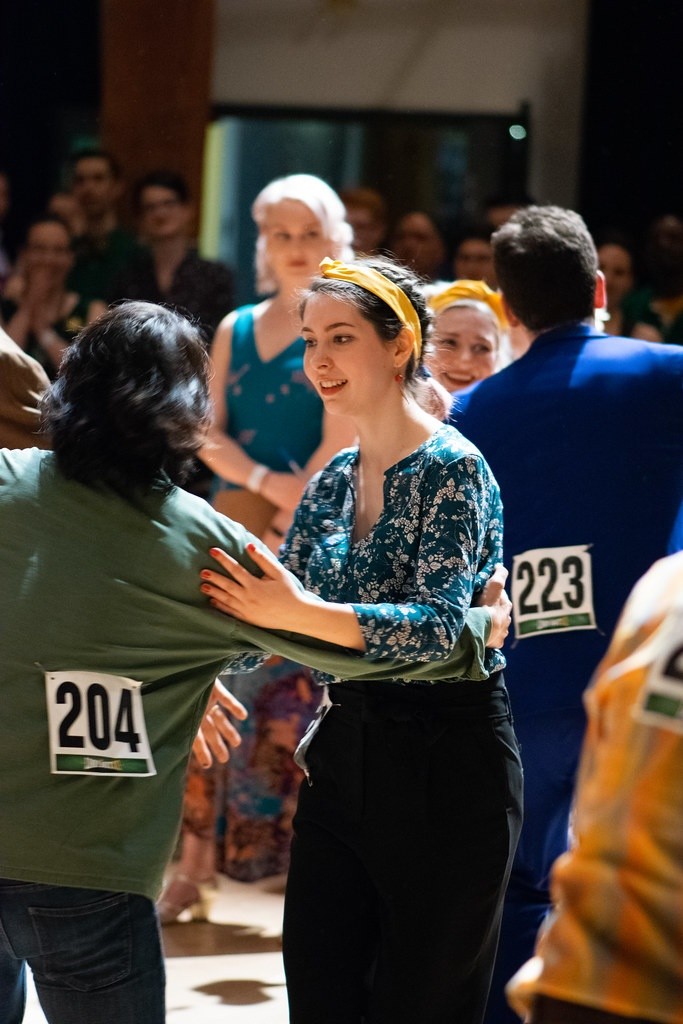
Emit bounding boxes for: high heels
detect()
[155,871,219,925]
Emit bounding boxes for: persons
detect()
[1,148,683,1024]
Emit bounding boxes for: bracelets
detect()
[247,464,268,495]
